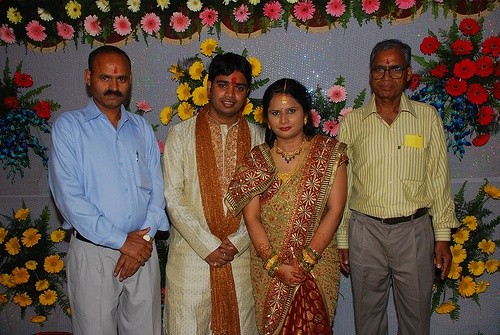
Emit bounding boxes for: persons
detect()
[336,39,461,335]
[238,78,349,335]
[48,45,170,335]
[163,52,266,335]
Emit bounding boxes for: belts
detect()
[364,207,428,224]
[72,227,93,243]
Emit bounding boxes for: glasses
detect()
[370,64,409,80]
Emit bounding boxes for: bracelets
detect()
[296,243,323,272]
[256,244,281,277]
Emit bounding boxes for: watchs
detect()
[141,234,152,242]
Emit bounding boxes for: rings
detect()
[214,262,218,267]
[138,257,143,261]
[223,253,226,259]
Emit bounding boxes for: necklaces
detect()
[277,138,305,163]
